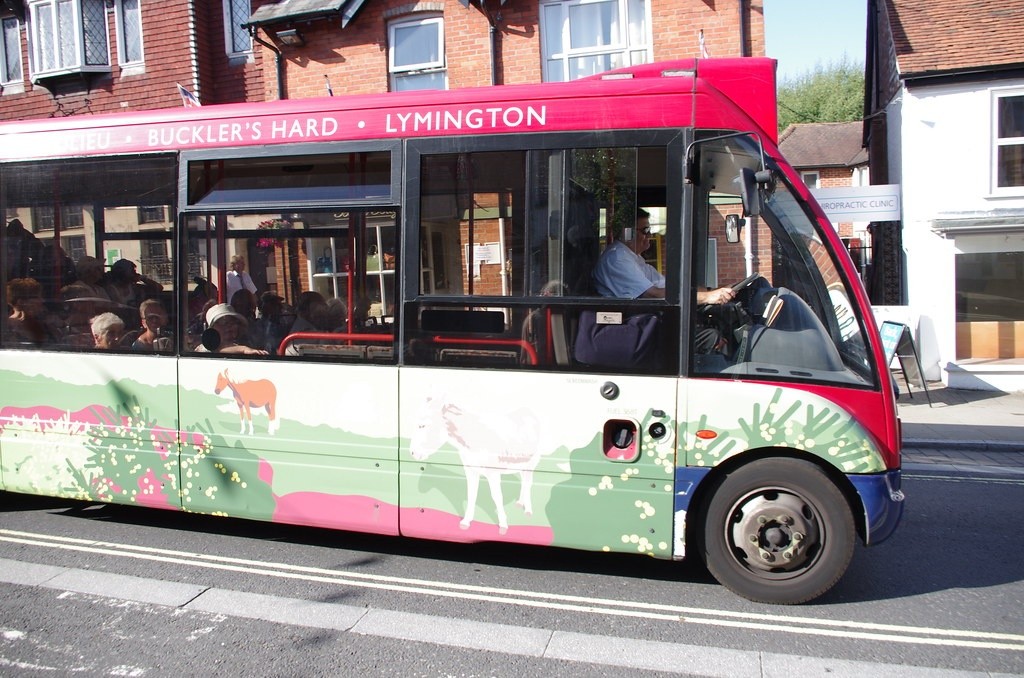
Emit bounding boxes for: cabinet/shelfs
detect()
[307,226,436,318]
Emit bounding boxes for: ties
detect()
[237,274,247,290]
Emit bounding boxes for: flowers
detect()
[257,220,291,248]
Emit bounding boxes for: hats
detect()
[75,255,107,275]
[205,306,249,337]
[258,292,285,314]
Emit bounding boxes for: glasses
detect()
[630,225,652,236]
[213,317,240,327]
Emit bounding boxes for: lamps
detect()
[275,28,305,49]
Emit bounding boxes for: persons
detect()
[188,276,285,355]
[132,298,174,352]
[71,256,112,312]
[520,280,571,365]
[226,255,258,319]
[58,285,98,346]
[106,259,163,323]
[285,291,371,357]
[591,201,736,356]
[88,313,132,350]
[7,219,76,347]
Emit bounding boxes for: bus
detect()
[1,55,906,604]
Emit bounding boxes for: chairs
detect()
[566,225,661,371]
